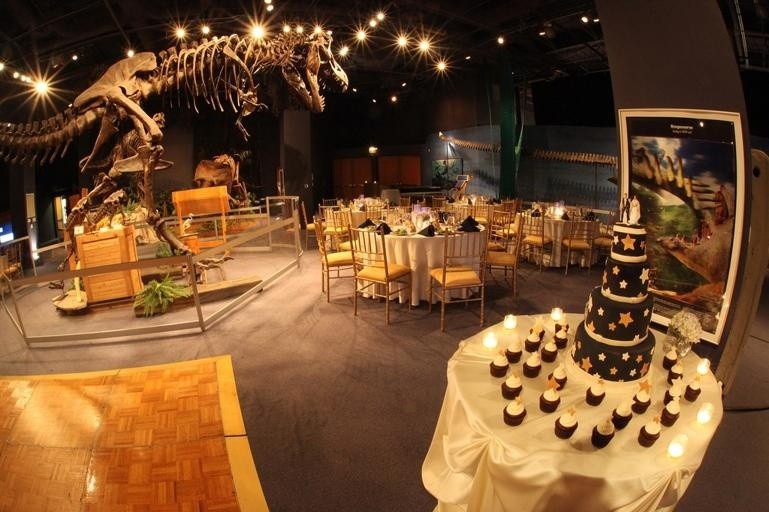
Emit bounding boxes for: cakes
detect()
[550,363,568,391]
[530,315,545,341]
[638,414,662,449]
[489,350,510,378]
[553,326,568,349]
[555,312,569,334]
[663,376,684,406]
[503,395,527,428]
[570,192,656,383]
[525,332,541,353]
[541,339,558,363]
[555,405,579,440]
[501,370,522,399]
[683,372,701,403]
[506,337,522,364]
[631,378,650,415]
[611,394,636,431]
[540,378,561,413]
[667,358,683,385]
[523,351,541,379]
[662,345,678,370]
[586,380,606,407]
[591,414,616,450]
[660,395,681,428]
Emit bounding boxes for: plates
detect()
[390,232,415,236]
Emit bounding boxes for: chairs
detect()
[347,224,412,324]
[331,209,354,278]
[565,212,597,275]
[432,196,447,213]
[318,204,348,253]
[493,204,517,248]
[429,227,490,331]
[594,207,620,266]
[301,201,326,247]
[399,196,410,214]
[312,215,353,303]
[489,210,512,282]
[365,203,382,219]
[518,209,553,273]
[0,242,25,288]
[323,198,338,213]
[487,216,527,298]
